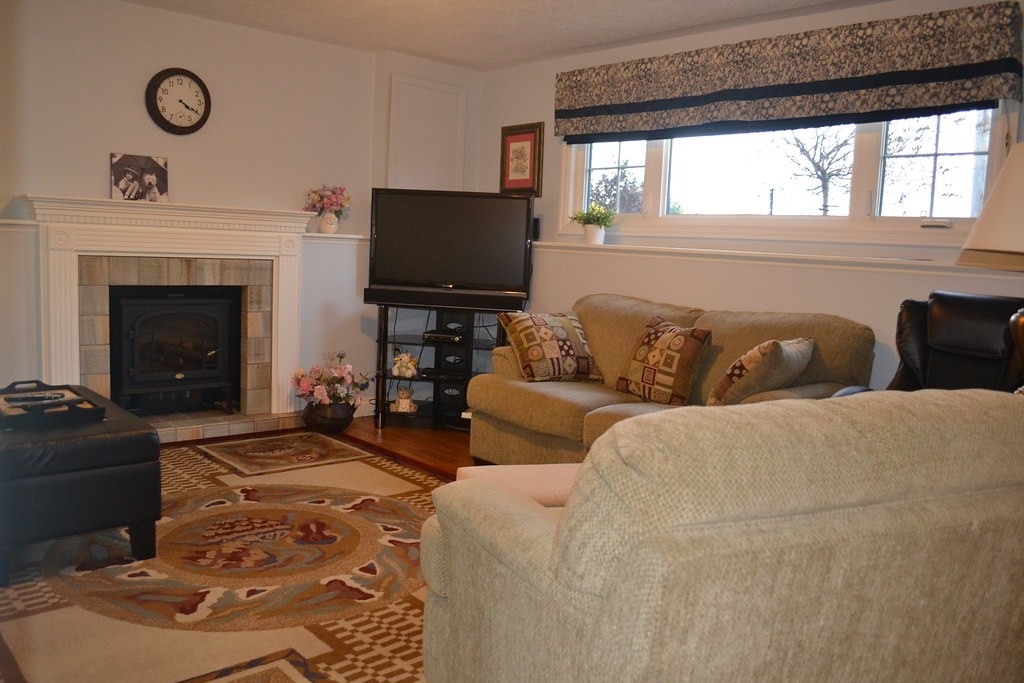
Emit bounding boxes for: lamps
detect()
[955,142,1024,394]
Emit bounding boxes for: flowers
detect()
[295,350,383,407]
[303,184,352,219]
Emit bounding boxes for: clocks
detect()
[144,67,212,135]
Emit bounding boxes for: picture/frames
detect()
[110,153,169,203]
[499,121,546,198]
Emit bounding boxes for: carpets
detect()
[0,423,458,682]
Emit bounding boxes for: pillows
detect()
[706,337,815,406]
[497,310,605,384]
[616,314,712,406]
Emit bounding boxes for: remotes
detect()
[4,393,66,402]
[21,396,87,412]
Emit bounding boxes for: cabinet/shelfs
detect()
[364,303,525,430]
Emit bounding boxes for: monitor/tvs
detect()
[369,187,534,311]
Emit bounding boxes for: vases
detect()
[317,215,339,234]
[302,401,355,434]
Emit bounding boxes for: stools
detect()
[0,382,162,587]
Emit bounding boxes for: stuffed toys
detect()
[390,386,418,412]
[392,353,417,377]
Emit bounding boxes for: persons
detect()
[118,167,142,201]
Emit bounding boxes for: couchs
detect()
[416,390,1024,683]
[884,289,1024,394]
[467,292,876,466]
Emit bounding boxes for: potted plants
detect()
[568,202,617,244]
